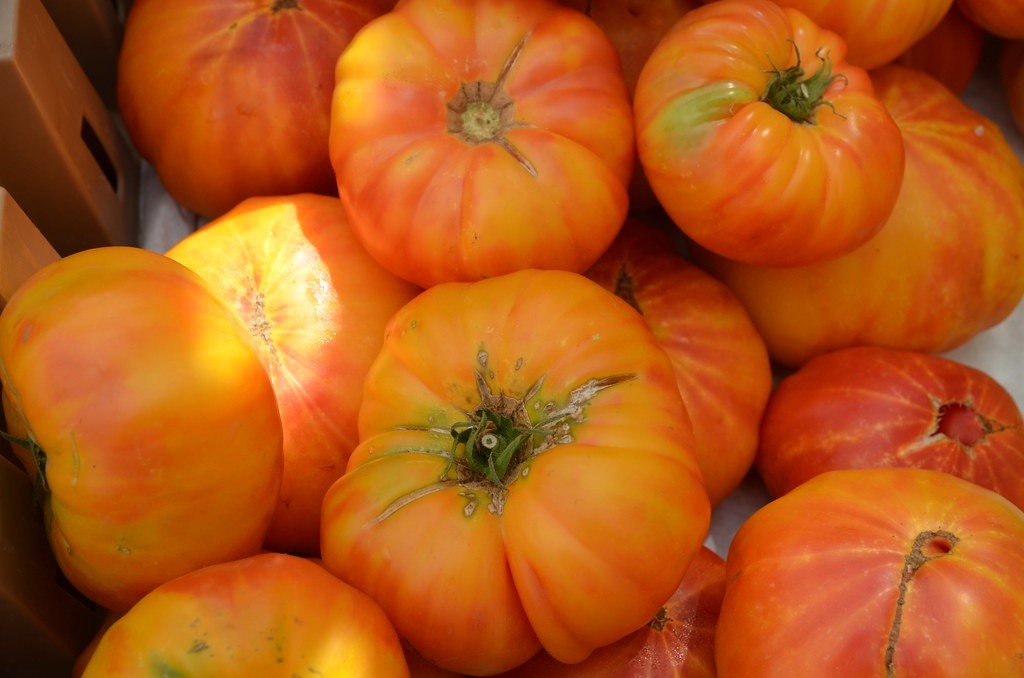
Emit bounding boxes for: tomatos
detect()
[0,0,1024,678]
